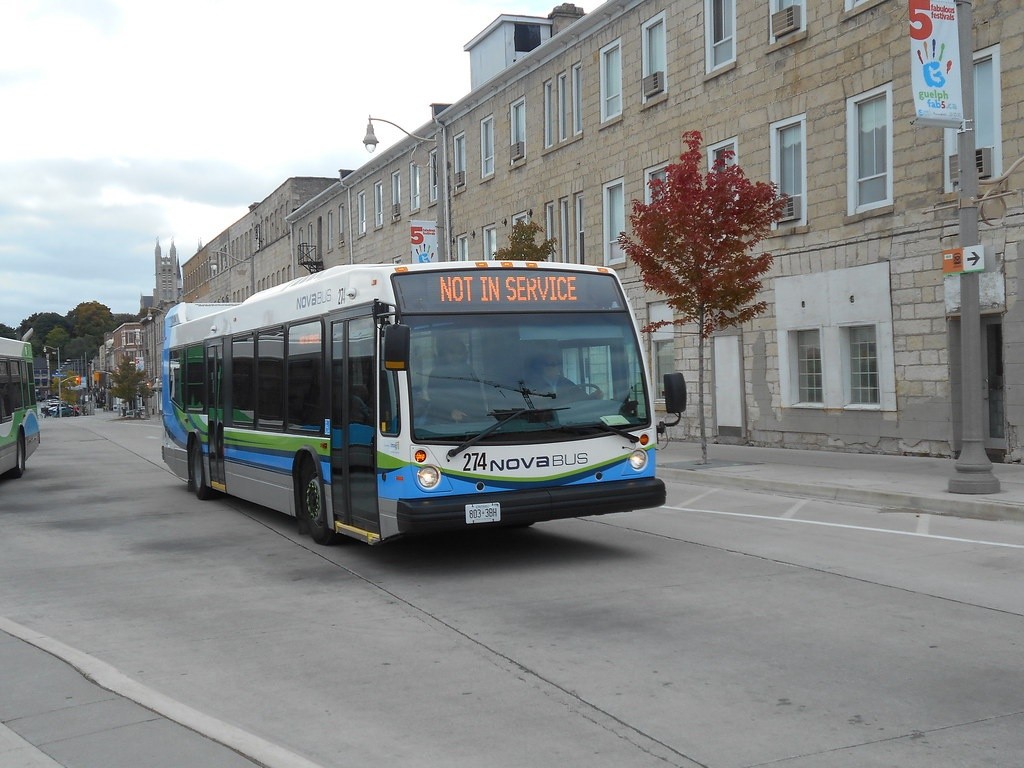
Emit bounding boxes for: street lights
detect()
[211,249,254,297]
[365,114,446,262]
[43,346,63,417]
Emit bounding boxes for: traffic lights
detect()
[76,376,81,385]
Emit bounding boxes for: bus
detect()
[159,259,687,546]
[0,336,40,478]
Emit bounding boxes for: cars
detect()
[41,394,79,418]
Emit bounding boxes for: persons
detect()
[524,356,604,413]
[42,398,129,419]
[350,382,372,423]
[424,332,488,426]
